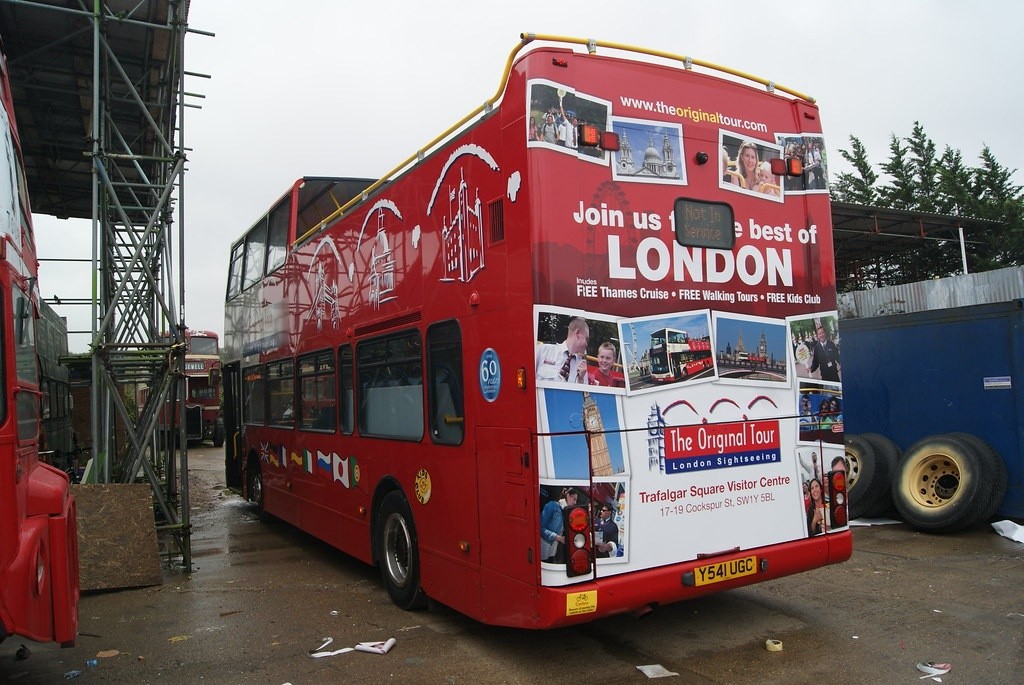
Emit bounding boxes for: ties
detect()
[558,350,576,382]
[573,127,576,145]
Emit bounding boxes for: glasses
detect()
[601,508,609,512]
[570,496,577,501]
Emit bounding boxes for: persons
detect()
[539,486,578,564]
[819,476,831,516]
[808,479,830,538]
[637,312,844,445]
[587,342,626,392]
[590,504,620,559]
[798,451,823,486]
[802,482,813,516]
[830,456,846,478]
[533,318,589,385]
[524,77,830,208]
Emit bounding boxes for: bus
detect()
[130,329,225,453]
[646,327,713,385]
[208,33,854,632]
